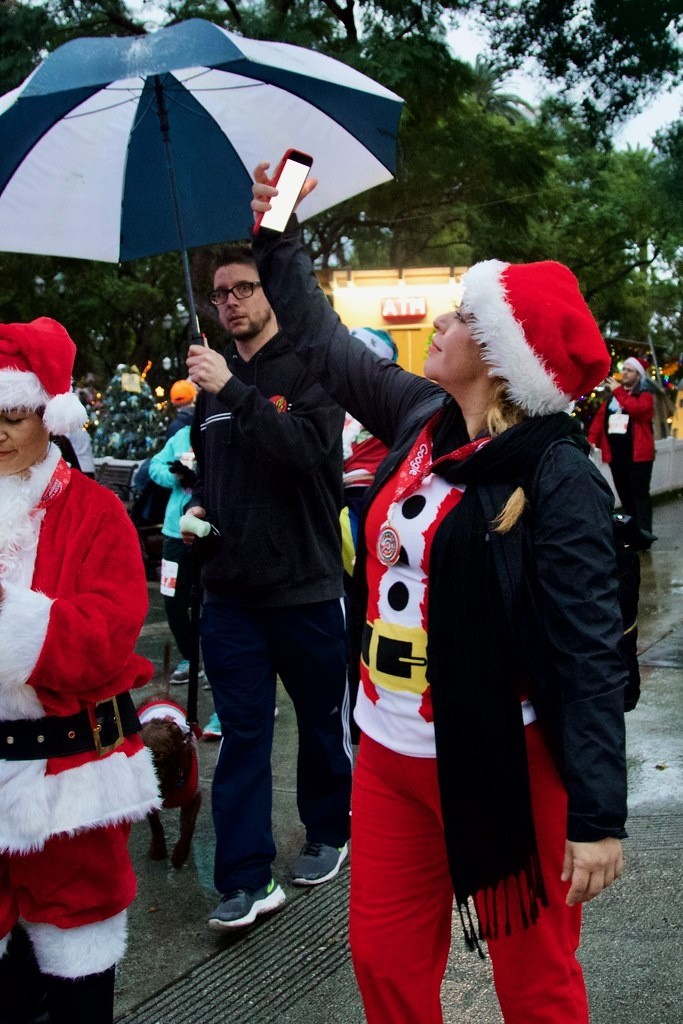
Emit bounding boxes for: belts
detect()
[0,692,142,761]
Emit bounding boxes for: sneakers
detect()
[169,660,204,684]
[205,877,286,930]
[202,674,211,689]
[201,706,279,736]
[287,841,349,884]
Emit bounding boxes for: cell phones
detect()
[252,148,314,242]
[606,367,613,379]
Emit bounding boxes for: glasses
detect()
[206,282,262,305]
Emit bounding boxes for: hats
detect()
[169,380,198,405]
[0,316,94,472]
[624,357,649,389]
[460,259,612,415]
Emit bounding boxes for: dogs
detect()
[136,642,203,870]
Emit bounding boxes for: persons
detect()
[585,356,658,550]
[131,383,281,740]
[182,245,350,928]
[249,164,630,1024]
[0,316,162,1024]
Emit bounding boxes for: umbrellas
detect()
[0,15,404,345]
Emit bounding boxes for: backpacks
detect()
[395,392,640,712]
